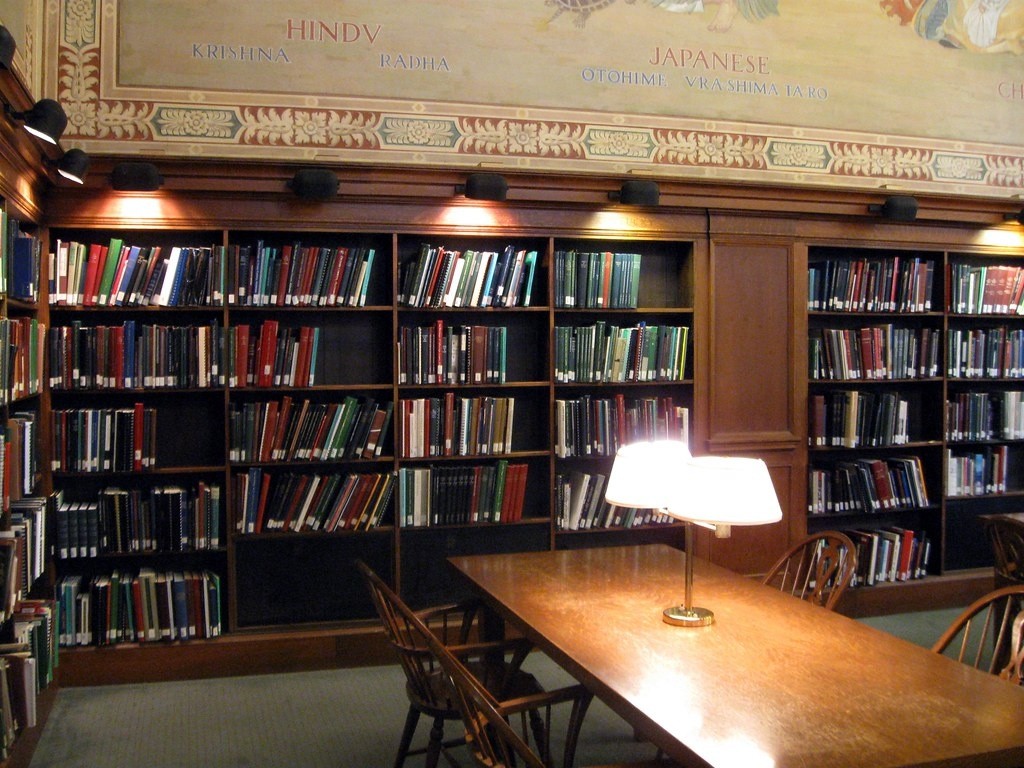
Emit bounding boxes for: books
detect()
[399,244,548,527]
[943,263,1023,501]
[802,258,942,591]
[553,249,696,533]
[58,234,222,649]
[0,194,58,768]
[229,237,398,536]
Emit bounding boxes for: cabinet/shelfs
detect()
[0,63,1024,768]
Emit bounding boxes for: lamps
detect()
[867,196,919,223]
[456,172,508,202]
[48,148,90,185]
[12,98,68,144]
[605,440,782,627]
[0,25,17,69]
[113,161,163,190]
[608,180,661,207]
[286,167,342,202]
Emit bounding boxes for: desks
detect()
[443,542,1024,768]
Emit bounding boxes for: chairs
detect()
[429,638,597,768]
[762,530,858,611]
[930,587,1024,686]
[354,559,553,768]
